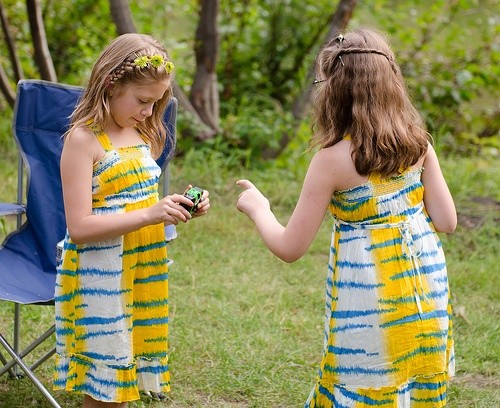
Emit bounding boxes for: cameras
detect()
[180,188,204,216]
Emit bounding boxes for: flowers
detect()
[134,53,175,74]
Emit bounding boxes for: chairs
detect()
[1,79,179,407]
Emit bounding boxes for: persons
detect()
[231,28,460,408]
[52,31,213,408]
